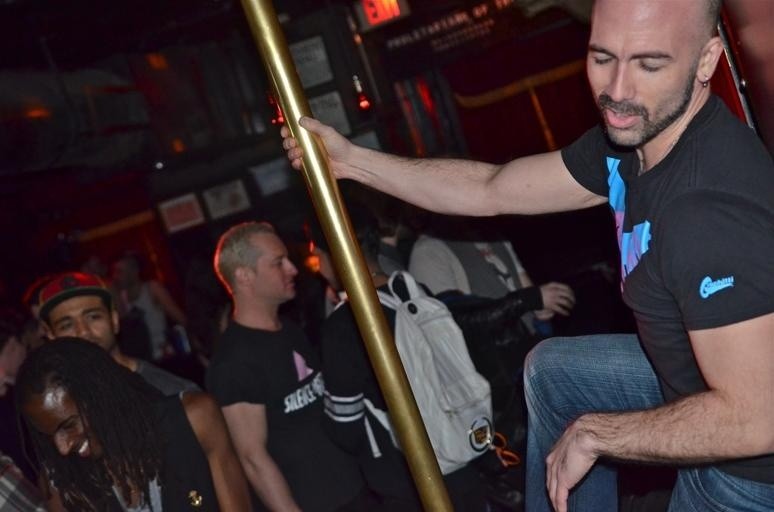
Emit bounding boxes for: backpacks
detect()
[337,269,494,479]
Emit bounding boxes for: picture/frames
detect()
[152,30,395,241]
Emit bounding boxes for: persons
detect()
[276,2,773,511]
[215,206,575,511]
[1,269,253,511]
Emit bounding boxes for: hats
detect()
[37,271,112,319]
